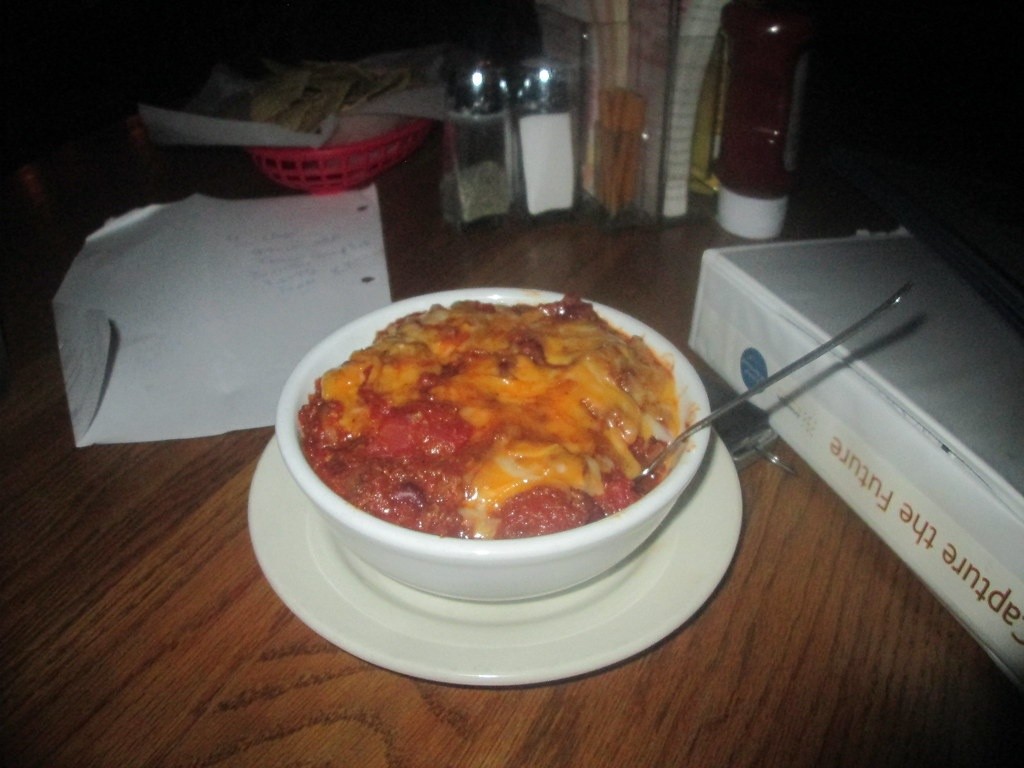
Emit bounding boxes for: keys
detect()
[699,373,781,474]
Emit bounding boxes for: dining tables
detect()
[0,109,1021,767]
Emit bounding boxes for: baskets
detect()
[246,118,432,192]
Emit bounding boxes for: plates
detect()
[247,421,745,689]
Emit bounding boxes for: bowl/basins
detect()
[277,288,714,606]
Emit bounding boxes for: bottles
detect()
[507,51,583,233]
[711,4,808,242]
[435,55,511,232]
